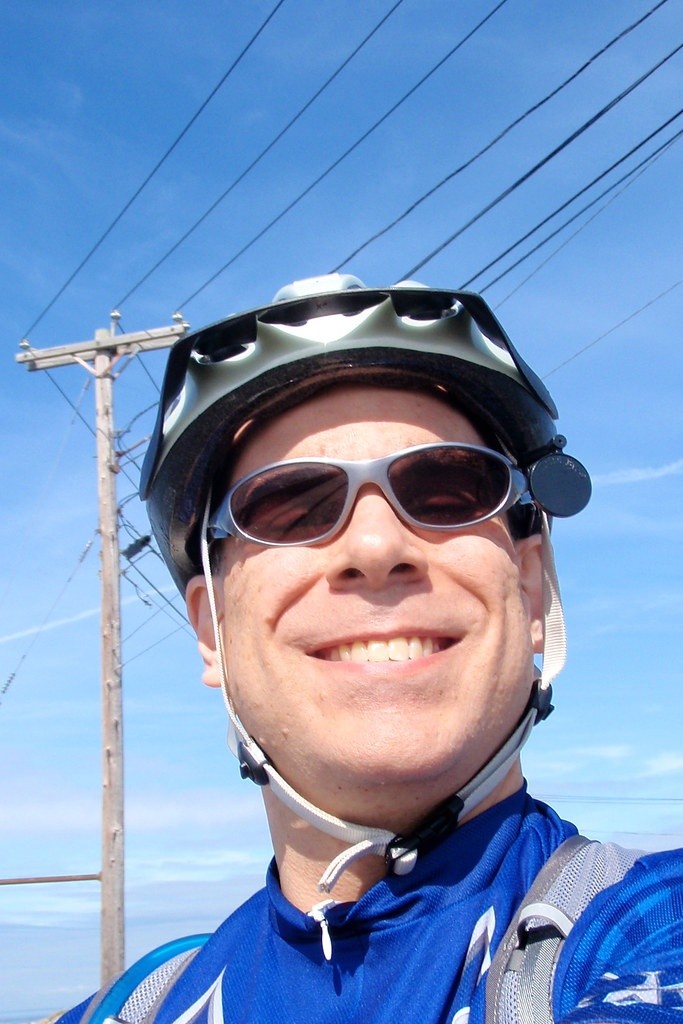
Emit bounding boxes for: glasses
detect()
[208,441,535,548]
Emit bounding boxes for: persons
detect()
[39,272,683,1024]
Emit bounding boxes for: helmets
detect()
[139,273,591,602]
